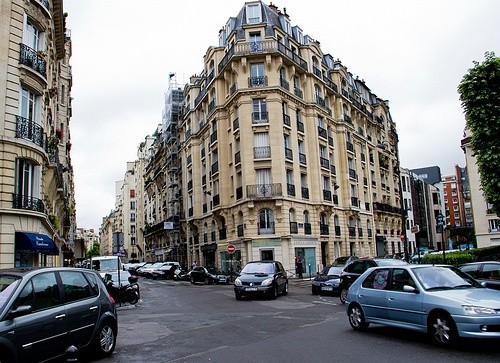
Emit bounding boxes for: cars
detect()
[312,251,500,349]
[78,256,137,299]
[122,262,180,279]
[191,266,229,285]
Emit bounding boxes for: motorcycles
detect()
[106,276,140,304]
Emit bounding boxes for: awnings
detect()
[15,232,59,256]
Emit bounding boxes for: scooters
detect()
[174,265,189,281]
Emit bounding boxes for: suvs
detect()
[234,261,289,300]
[0,267,118,363]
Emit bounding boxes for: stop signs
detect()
[227,244,235,253]
[400,232,404,241]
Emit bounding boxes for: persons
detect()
[296,256,304,279]
[192,260,198,269]
[383,251,396,259]
